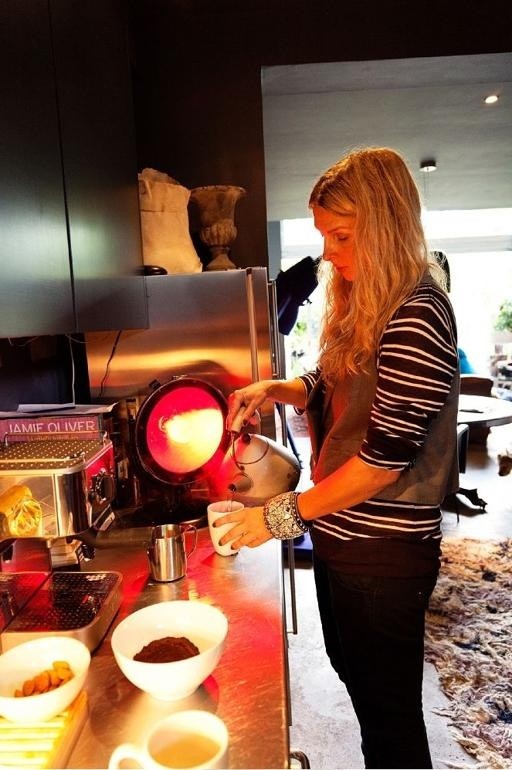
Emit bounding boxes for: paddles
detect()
[420,538,512,770]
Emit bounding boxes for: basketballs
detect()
[12,660,75,697]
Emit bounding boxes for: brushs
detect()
[0,429,123,655]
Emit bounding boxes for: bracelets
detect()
[110,601,228,700]
[4,636,91,724]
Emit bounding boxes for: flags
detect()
[185,186,246,271]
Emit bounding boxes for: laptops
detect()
[0,483,45,537]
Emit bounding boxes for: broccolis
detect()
[85,268,287,503]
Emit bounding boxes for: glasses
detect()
[419,159,451,292]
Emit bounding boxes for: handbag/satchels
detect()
[262,490,314,541]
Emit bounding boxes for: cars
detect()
[108,710,229,769]
[207,500,244,556]
[143,524,197,582]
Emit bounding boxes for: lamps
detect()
[459,374,494,396]
[443,423,488,514]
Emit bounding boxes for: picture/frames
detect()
[454,393,512,446]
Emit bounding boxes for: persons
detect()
[211,146,462,770]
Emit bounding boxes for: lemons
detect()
[215,406,301,508]
